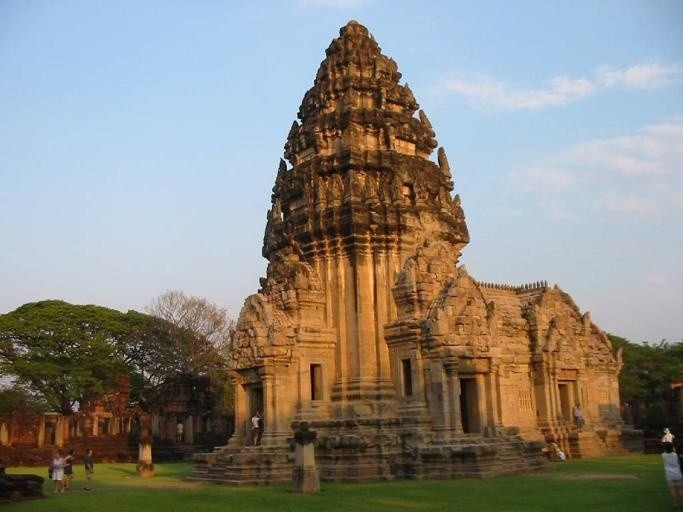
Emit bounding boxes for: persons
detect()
[252,409,264,445]
[48,448,80,497]
[659,426,683,508]
[574,403,583,432]
[85,448,94,492]
[177,423,185,441]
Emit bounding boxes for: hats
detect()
[664,428,670,434]
